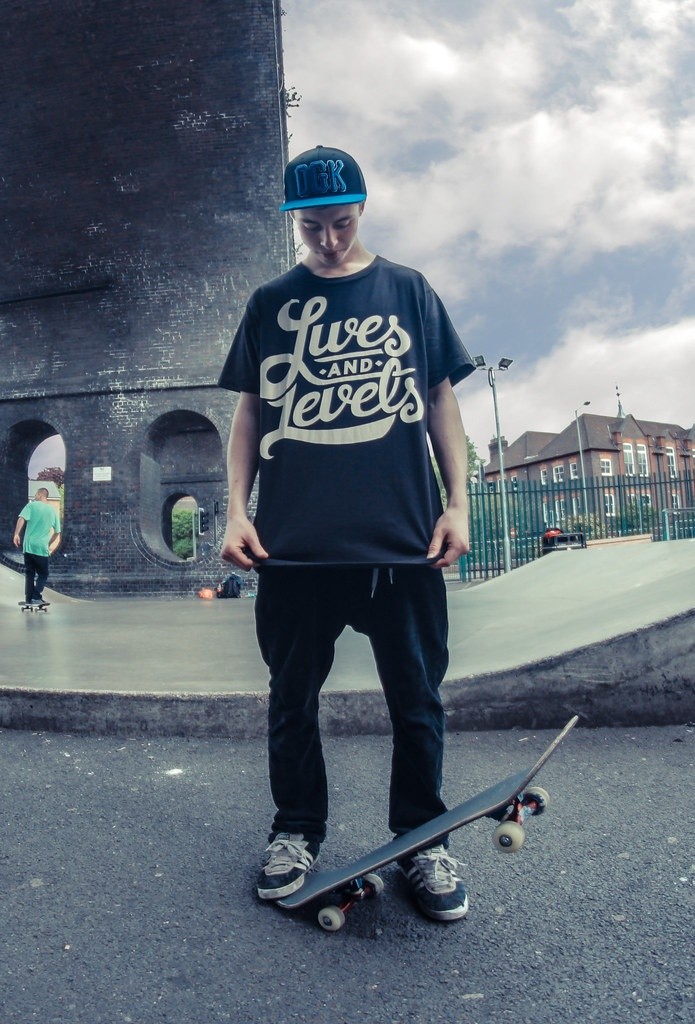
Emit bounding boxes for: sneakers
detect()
[256,832,321,899]
[397,843,469,921]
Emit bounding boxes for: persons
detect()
[13,488,62,605]
[220,143,473,924]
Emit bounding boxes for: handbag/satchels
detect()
[198,589,212,599]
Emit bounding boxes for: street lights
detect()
[574,400,592,523]
[472,355,514,576]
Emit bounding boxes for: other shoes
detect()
[25,600,30,606]
[31,599,46,606]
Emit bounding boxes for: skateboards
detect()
[274,714,581,932]
[18,601,50,613]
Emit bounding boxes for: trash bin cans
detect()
[542,533,586,557]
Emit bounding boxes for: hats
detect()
[279,145,367,212]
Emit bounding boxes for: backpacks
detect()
[217,573,242,599]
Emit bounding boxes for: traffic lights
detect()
[198,507,209,536]
